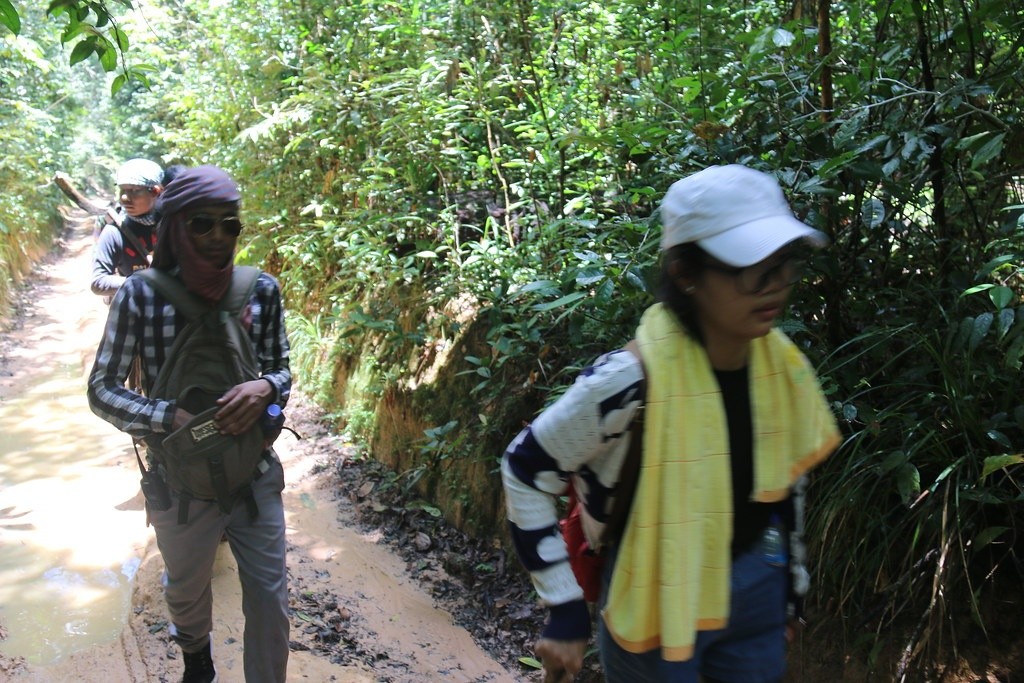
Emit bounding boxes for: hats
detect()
[117,158,163,187]
[156,165,243,216]
[660,165,829,267]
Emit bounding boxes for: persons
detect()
[87,165,293,683]
[91,158,188,307]
[502,164,842,683]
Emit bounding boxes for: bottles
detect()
[261,404,285,448]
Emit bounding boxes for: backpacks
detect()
[131,266,300,524]
[560,339,646,601]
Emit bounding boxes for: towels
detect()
[603,299,843,662]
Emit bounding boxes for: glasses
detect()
[186,215,244,239]
[715,253,811,294]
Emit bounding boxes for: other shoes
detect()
[182,640,216,683]
